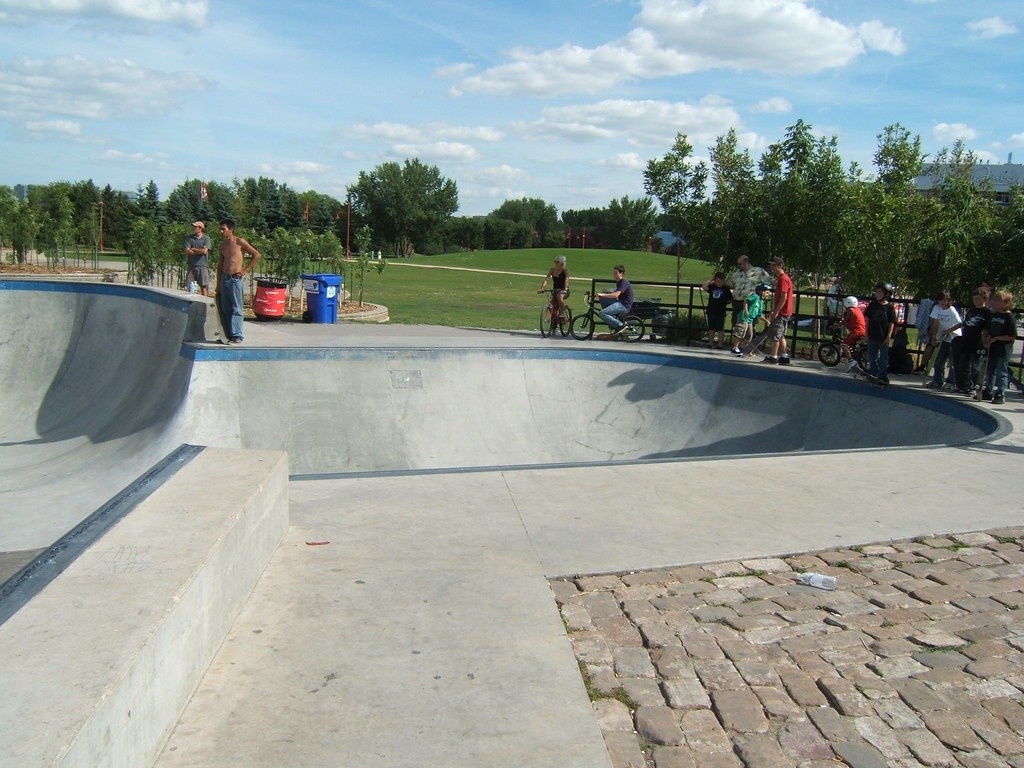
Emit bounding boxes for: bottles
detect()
[795,573,837,591]
[188,281,198,295]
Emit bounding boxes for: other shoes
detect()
[913,367,926,374]
[217,336,234,342]
[972,388,993,402]
[941,383,956,389]
[992,391,1005,403]
[234,337,244,342]
[926,381,942,389]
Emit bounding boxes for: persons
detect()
[829,282,897,385]
[596,265,635,336]
[215,219,261,344]
[184,221,210,296]
[702,272,733,349]
[725,255,777,357]
[540,255,570,336]
[760,257,794,365]
[912,287,1017,404]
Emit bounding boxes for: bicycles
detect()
[536,290,574,339]
[817,325,881,373]
[569,291,646,342]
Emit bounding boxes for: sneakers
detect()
[612,323,628,336]
[759,356,779,366]
[731,347,743,355]
[745,353,755,357]
[777,356,790,366]
[846,360,858,372]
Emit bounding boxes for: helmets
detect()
[874,281,893,296]
[843,296,858,308]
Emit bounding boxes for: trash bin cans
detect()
[252,276,290,321]
[299,272,343,324]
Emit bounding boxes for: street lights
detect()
[303,203,309,228]
[334,202,352,260]
[98,201,108,252]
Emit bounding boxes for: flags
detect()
[201,181,208,201]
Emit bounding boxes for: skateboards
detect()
[1008,371,1024,396]
[737,325,769,358]
[855,364,890,386]
[973,342,990,401]
[921,333,948,387]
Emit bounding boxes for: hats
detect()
[192,221,204,228]
[758,282,776,293]
[767,256,785,265]
[554,255,567,264]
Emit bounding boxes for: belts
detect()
[222,271,238,278]
